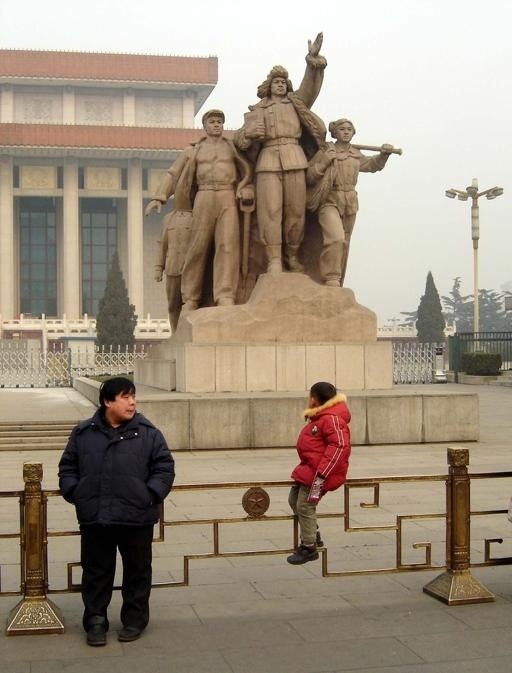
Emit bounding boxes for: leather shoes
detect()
[118,626,142,642]
[86,623,107,646]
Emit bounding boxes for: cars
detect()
[434,370,447,383]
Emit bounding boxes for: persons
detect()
[287,382,351,565]
[153,209,192,335]
[57,377,175,646]
[232,32,327,273]
[144,109,254,312]
[304,119,393,284]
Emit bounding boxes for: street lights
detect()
[445,177,504,351]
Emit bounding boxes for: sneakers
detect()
[287,531,323,565]
